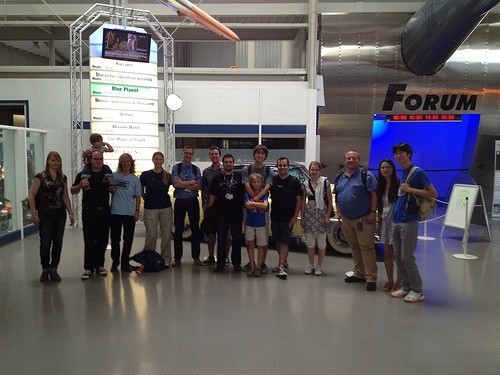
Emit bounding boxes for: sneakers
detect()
[81,270,92,279]
[403,291,425,303]
[95,266,107,274]
[392,286,409,297]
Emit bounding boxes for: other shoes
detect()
[194,258,201,266]
[254,268,262,275]
[262,264,269,274]
[366,282,377,292]
[40,272,49,281]
[121,265,133,272]
[315,264,323,275]
[345,276,364,283]
[108,185,116,191]
[225,257,231,266]
[272,263,289,272]
[305,264,315,274]
[201,256,215,266]
[50,272,62,281]
[233,265,243,272]
[277,264,288,277]
[138,265,146,272]
[242,263,256,272]
[84,185,91,191]
[247,268,254,276]
[171,260,182,267]
[213,265,225,272]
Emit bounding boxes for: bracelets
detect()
[370,210,376,214]
[301,219,304,221]
[68,212,74,215]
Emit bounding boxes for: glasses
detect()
[92,157,104,161]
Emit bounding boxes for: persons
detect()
[109,152,141,272]
[242,145,273,276]
[140,146,202,267]
[300,161,332,276]
[334,150,378,291]
[390,143,438,302]
[82,133,115,190]
[270,157,304,278]
[29,151,75,281]
[70,150,112,279]
[201,146,243,272]
[376,159,401,292]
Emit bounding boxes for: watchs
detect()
[136,209,140,212]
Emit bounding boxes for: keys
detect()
[358,222,363,231]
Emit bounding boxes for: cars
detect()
[169,158,380,258]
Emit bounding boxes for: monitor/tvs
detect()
[102,28,151,63]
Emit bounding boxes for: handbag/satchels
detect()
[323,180,335,217]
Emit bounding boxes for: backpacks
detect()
[405,166,436,221]
